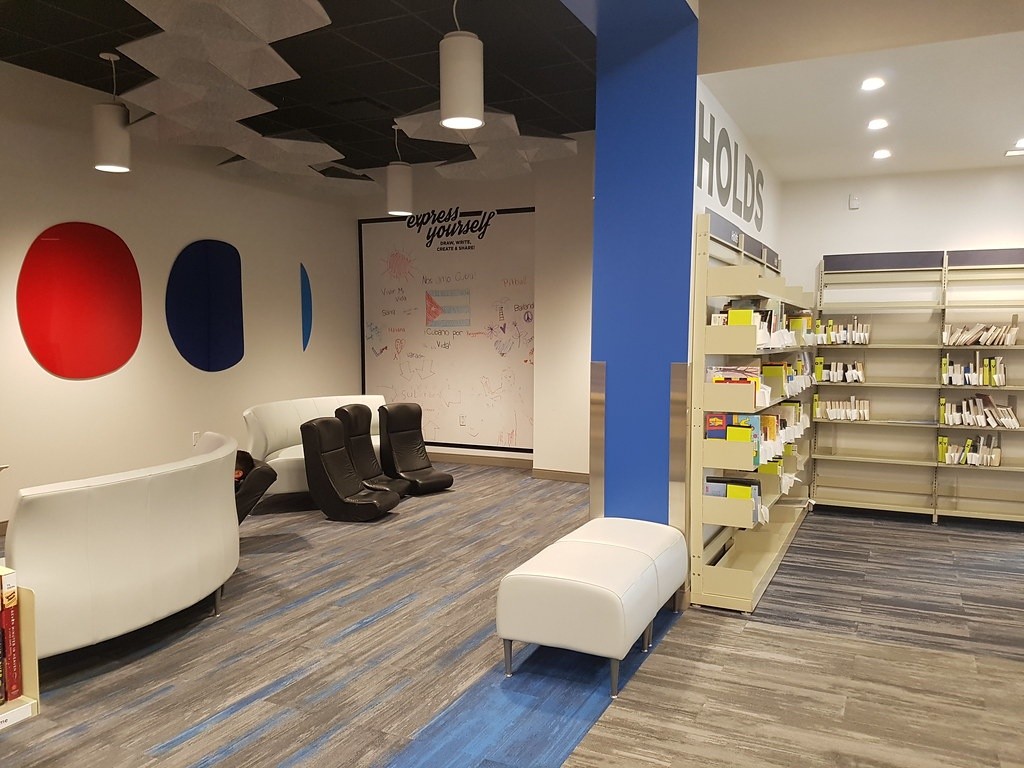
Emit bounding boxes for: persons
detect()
[234,450,255,494]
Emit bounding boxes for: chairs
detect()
[377,402,453,494]
[335,403,411,499]
[234,459,278,525]
[299,417,400,522]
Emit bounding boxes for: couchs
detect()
[0,430,246,662]
[244,389,393,494]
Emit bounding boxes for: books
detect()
[0,565,24,707]
[783,442,801,460]
[938,392,1021,430]
[937,434,1001,467]
[942,351,1007,387]
[704,352,866,406]
[705,476,769,525]
[711,299,872,349]
[813,394,870,422]
[758,456,802,495]
[943,322,1020,346]
[706,399,811,465]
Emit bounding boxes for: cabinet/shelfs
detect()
[809,249,1024,524]
[692,214,816,615]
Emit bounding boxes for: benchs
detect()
[494,515,693,698]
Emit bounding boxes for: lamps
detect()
[92,52,130,170]
[439,1,485,129]
[388,125,412,217]
